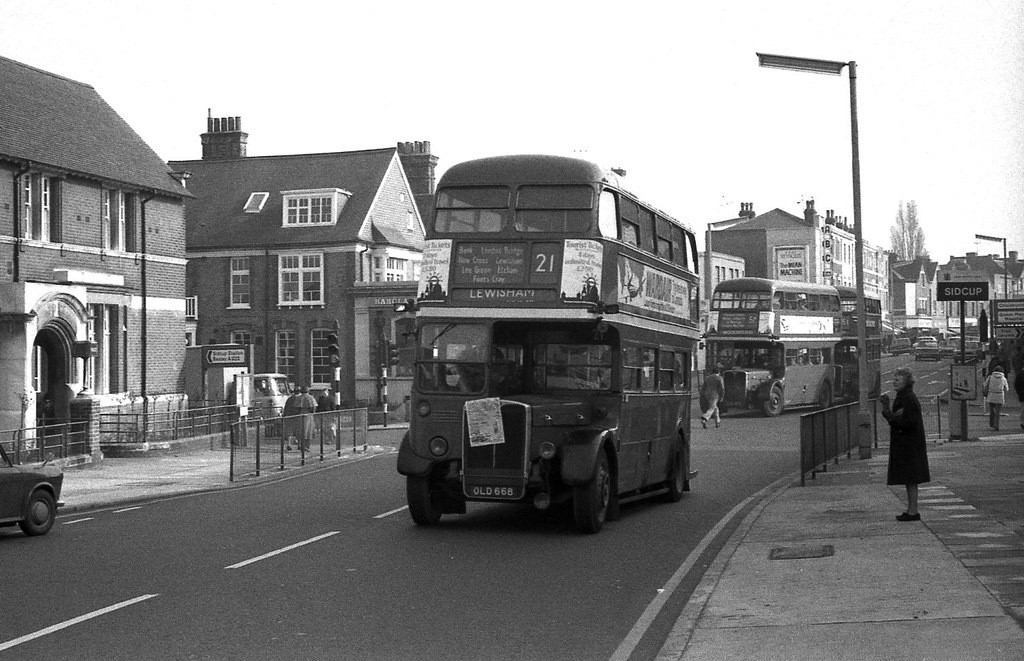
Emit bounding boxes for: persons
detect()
[878,366,931,522]
[982,366,1010,431]
[698,367,726,429]
[291,386,319,452]
[315,387,339,445]
[281,386,301,451]
[1013,361,1024,429]
[982,334,1024,383]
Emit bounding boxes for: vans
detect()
[250,373,298,425]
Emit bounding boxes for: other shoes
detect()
[701,419,721,428]
[896,512,921,521]
[286,446,310,452]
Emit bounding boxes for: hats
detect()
[293,386,309,394]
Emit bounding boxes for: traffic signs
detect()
[994,326,1024,341]
[992,300,1024,325]
[205,348,246,364]
[935,282,990,302]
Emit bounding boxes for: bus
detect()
[393,154,703,535]
[698,278,882,417]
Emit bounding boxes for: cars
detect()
[889,333,992,365]
[0,443,67,535]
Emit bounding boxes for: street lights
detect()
[975,233,1009,300]
[756,46,874,462]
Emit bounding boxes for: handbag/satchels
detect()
[983,385,989,397]
